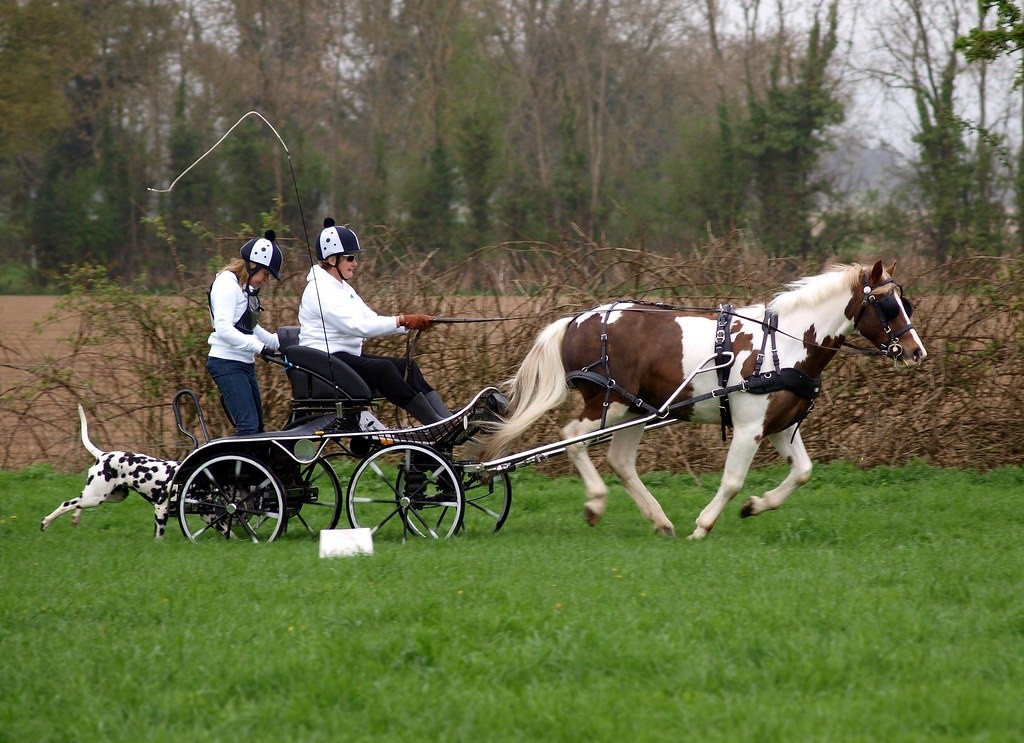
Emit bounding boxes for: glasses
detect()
[341,255,355,262]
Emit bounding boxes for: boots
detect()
[405,391,461,440]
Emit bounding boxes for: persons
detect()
[297,217,461,439]
[205,230,284,436]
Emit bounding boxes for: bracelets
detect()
[399,313,406,327]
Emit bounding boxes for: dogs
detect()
[40,390,188,546]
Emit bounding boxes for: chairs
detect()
[265,326,388,413]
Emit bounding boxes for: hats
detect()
[316,217,366,260]
[241,230,284,281]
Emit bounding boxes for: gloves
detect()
[399,315,436,331]
[260,345,275,363]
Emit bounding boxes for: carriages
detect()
[167,257,926,541]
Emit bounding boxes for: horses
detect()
[468,257,930,548]
